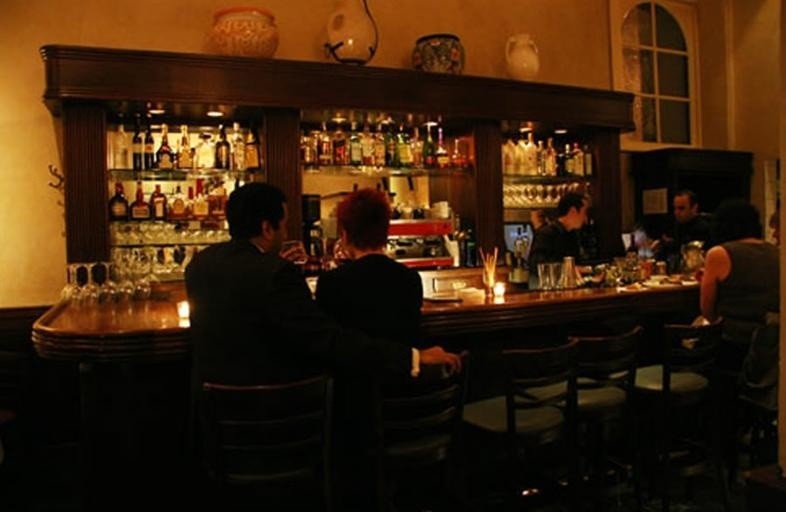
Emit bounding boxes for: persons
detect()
[769,212,780,247]
[527,190,605,276]
[183,183,463,431]
[316,187,423,417]
[665,189,708,251]
[700,199,779,373]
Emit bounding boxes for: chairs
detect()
[522,325,643,512]
[461,336,581,512]
[723,325,779,494]
[607,315,733,512]
[365,349,468,512]
[197,372,332,512]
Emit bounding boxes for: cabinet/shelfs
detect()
[30,270,702,459]
[629,147,753,263]
[41,45,636,286]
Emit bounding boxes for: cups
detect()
[117,260,133,302]
[60,262,83,311]
[81,262,99,308]
[133,256,155,298]
[563,256,578,289]
[536,262,554,291]
[97,261,116,304]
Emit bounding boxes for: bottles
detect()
[298,112,473,170]
[131,130,141,171]
[156,125,176,168]
[245,120,260,172]
[216,124,230,168]
[109,183,128,220]
[229,122,245,171]
[176,126,192,169]
[169,186,190,217]
[498,131,594,179]
[113,123,129,169]
[145,131,153,171]
[513,227,525,256]
[151,185,167,221]
[130,181,151,221]
